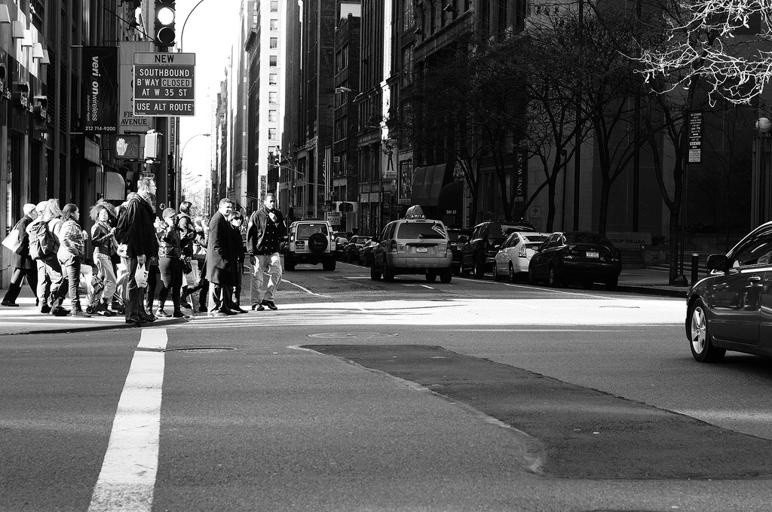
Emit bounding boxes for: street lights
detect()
[255,162,308,221]
[753,117,771,226]
[178,132,212,166]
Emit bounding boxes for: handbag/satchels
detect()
[186,260,201,286]
[83,237,93,263]
[182,257,192,274]
[2,229,23,253]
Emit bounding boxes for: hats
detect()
[162,208,177,220]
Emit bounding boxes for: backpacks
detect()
[114,199,148,246]
[28,217,57,259]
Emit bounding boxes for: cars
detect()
[686,220,772,363]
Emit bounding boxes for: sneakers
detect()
[2,285,286,323]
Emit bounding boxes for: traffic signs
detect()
[135,51,196,116]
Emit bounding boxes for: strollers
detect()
[76,267,129,316]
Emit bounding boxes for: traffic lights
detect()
[114,134,139,162]
[155,0,176,46]
[144,131,163,164]
